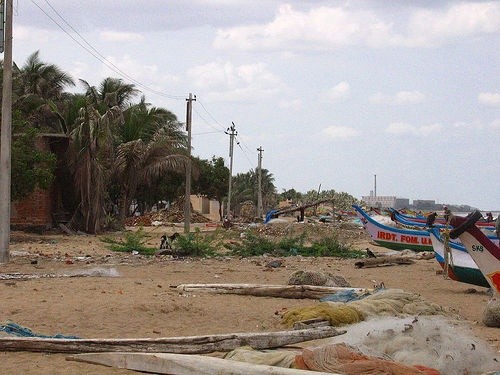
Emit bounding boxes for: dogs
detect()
[159,232,180,249]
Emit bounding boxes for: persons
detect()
[160,232,167,248]
[222,216,225,227]
[426,212,438,228]
[444,207,451,223]
[390,210,397,224]
[486,213,494,223]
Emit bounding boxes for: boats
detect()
[349,205,499,253]
[382,202,500,298]
